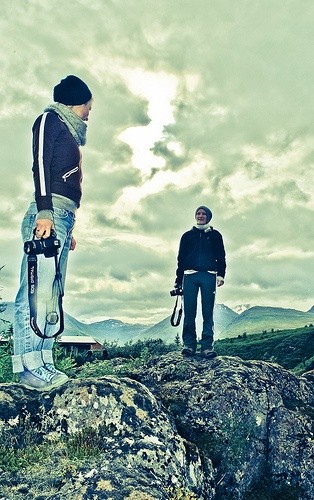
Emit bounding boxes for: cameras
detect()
[24,227,60,257]
[170,286,183,296]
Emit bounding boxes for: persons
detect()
[10,75,94,393]
[175,206,228,359]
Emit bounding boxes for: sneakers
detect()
[15,364,68,391]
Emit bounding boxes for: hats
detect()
[54,75,92,105]
[195,206,213,224]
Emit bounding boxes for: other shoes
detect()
[182,348,196,355]
[200,349,217,358]
